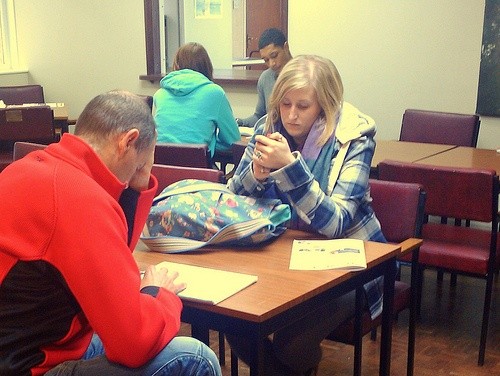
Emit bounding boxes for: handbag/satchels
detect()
[139,179,292,254]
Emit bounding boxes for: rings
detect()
[254,150,263,160]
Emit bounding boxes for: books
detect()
[289,238,367,273]
[138,260,259,306]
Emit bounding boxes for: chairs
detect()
[0,81,497,375]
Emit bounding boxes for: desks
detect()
[7,103,69,134]
[228,124,459,186]
[409,145,499,187]
[132,228,403,376]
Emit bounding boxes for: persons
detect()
[151,41,242,169]
[0,88,222,376]
[226,54,388,376]
[236,28,294,129]
